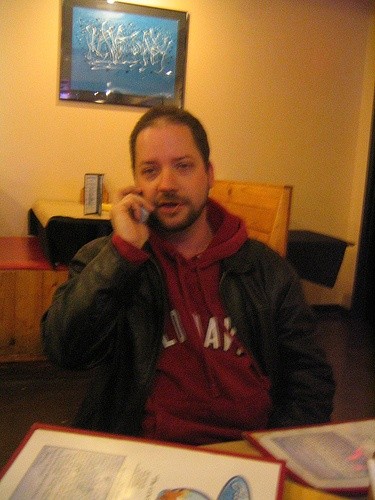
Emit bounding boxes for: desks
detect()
[27,198,116,268]
[285,229,355,309]
[186,440,375,500]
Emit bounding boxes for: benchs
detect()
[208,178,284,246]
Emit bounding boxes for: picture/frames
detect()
[59,0,191,111]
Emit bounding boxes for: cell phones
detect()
[130,207,149,223]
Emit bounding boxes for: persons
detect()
[39,105,337,453]
